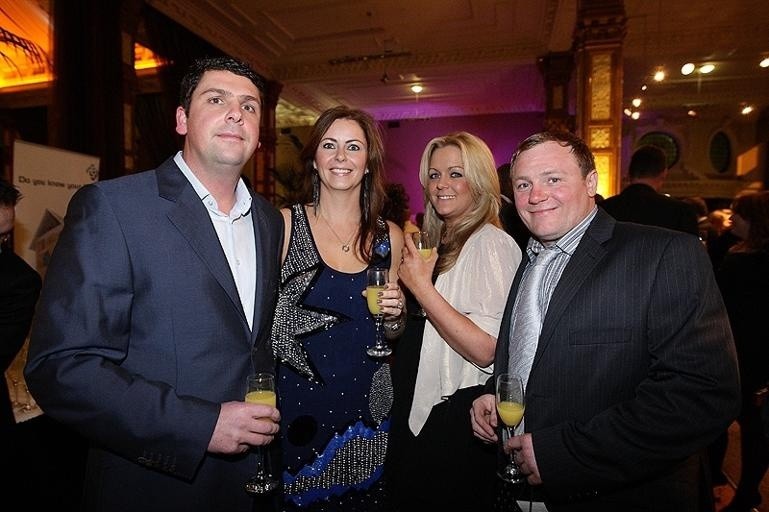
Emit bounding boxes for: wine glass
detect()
[241,371,280,495]
[492,372,527,486]
[364,266,393,358]
[410,230,433,318]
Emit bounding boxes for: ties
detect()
[503,247,560,448]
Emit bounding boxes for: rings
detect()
[397,298,404,308]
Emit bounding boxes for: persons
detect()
[394,131,522,511]
[0,177,43,432]
[469,129,742,512]
[263,106,408,512]
[23,61,283,512]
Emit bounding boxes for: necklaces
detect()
[318,206,363,252]
